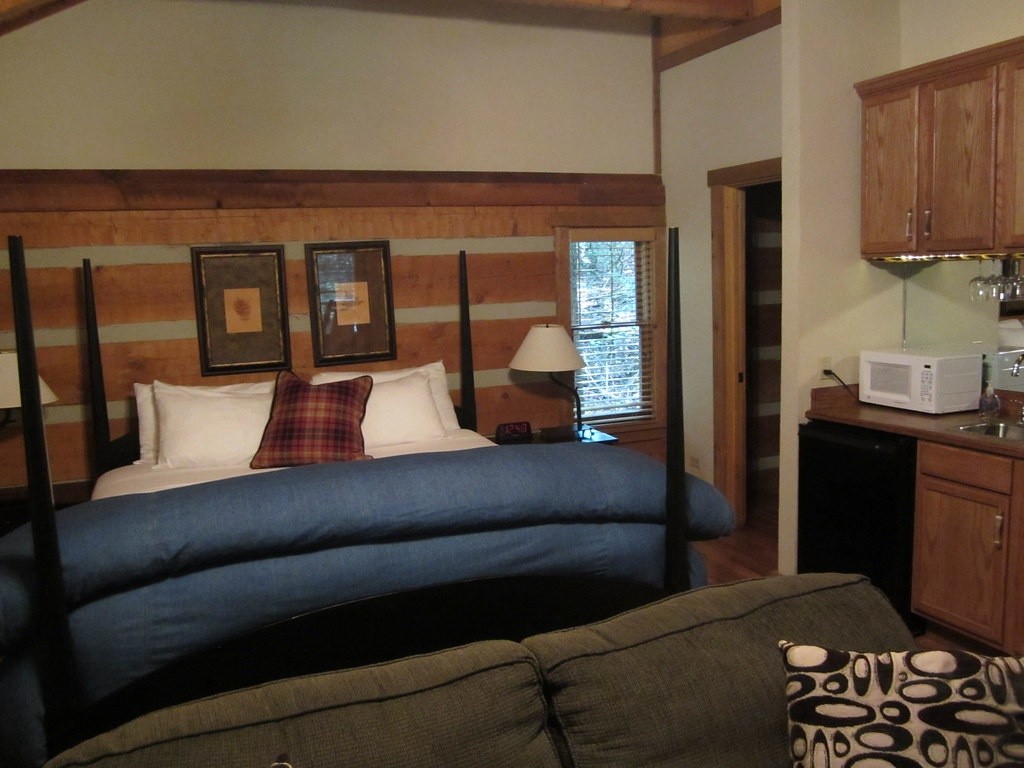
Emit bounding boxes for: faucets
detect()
[1011,349,1024,427]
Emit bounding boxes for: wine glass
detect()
[987,260,998,299]
[1005,259,1024,302]
[992,259,1011,301]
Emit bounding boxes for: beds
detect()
[0,223,736,767]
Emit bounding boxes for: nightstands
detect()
[489,422,618,451]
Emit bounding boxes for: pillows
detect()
[780,639,1024,767]
[132,379,276,465]
[249,369,375,470]
[152,379,278,470]
[44,639,562,768]
[310,361,462,432]
[522,571,926,768]
[360,371,445,448]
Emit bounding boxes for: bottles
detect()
[969,257,990,304]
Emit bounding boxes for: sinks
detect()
[946,420,1024,444]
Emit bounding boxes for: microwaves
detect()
[859,348,982,413]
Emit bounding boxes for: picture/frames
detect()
[189,244,291,378]
[303,240,398,368]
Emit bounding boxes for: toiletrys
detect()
[979,380,1001,419]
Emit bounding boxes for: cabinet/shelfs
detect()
[911,437,1023,658]
[852,40,1007,258]
[999,34,1024,255]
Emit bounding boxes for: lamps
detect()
[509,322,588,435]
[0,350,58,428]
